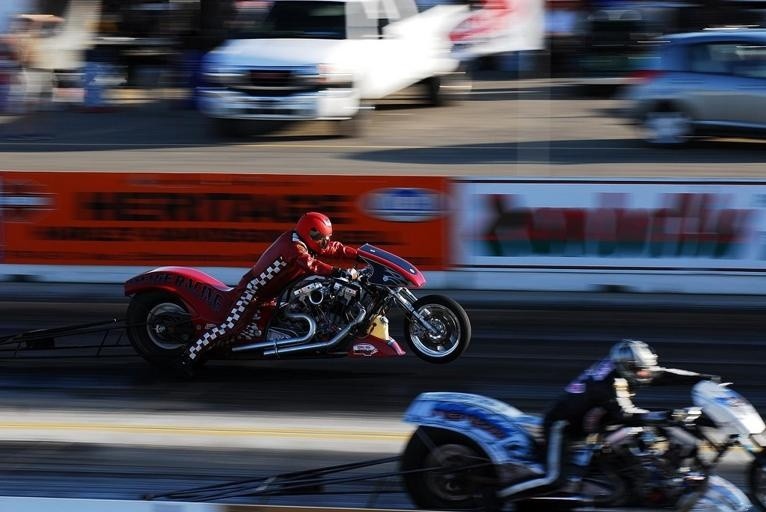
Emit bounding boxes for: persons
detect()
[484,337,725,509]
[180,213,365,366]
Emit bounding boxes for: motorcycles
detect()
[397,375,766,512]
[124,243,472,369]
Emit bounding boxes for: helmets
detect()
[296,212,333,255]
[612,340,658,384]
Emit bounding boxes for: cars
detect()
[621,22,766,152]
[195,1,455,122]
